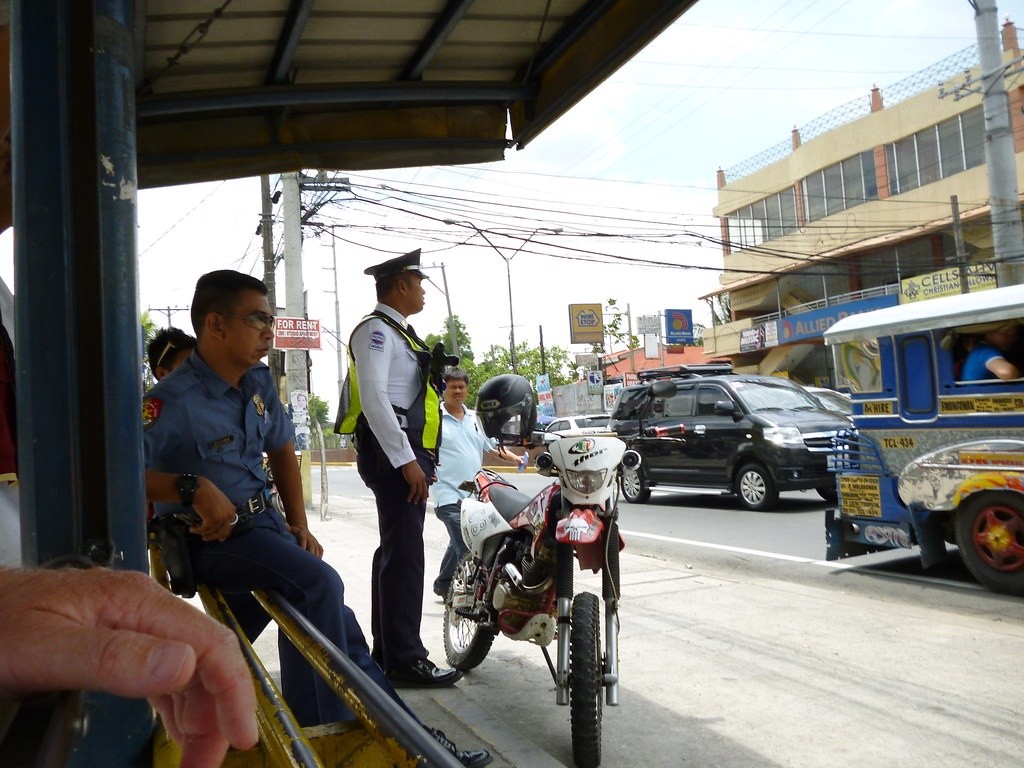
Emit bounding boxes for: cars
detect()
[544,413,612,442]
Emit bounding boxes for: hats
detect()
[365,247,430,281]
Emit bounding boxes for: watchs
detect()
[177,470,198,508]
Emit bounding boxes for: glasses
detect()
[222,311,275,331]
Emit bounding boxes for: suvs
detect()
[607,363,859,512]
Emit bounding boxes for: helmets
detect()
[473,375,536,444]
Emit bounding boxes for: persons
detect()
[335,249,467,689]
[296,394,308,409]
[141,271,493,768]
[430,366,528,605]
[959,325,1020,382]
[148,329,198,379]
[2,564,257,768]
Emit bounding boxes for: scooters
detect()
[824,283,1024,596]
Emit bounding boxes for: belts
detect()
[234,490,269,519]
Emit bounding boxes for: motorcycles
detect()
[442,380,676,767]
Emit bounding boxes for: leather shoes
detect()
[424,725,491,767]
[386,656,460,687]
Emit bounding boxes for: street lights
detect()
[445,218,563,374]
